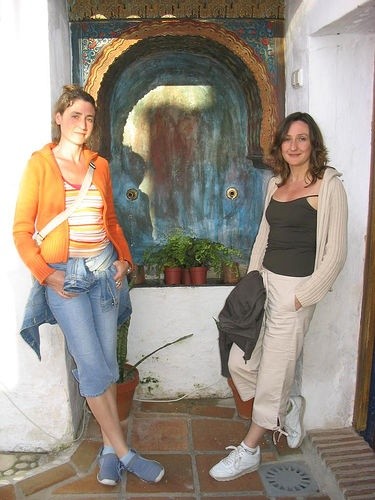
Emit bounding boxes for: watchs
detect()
[122,259,131,275]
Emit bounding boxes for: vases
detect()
[228,378,254,419]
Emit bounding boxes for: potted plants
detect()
[116,316,193,421]
[128,233,244,286]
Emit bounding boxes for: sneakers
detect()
[208,446,262,482]
[283,395,306,449]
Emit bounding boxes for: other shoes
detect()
[97,446,122,486]
[123,448,165,484]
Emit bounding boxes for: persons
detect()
[209,112,348,482]
[13,84,166,487]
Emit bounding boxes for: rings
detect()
[119,282,121,285]
[116,281,118,285]
